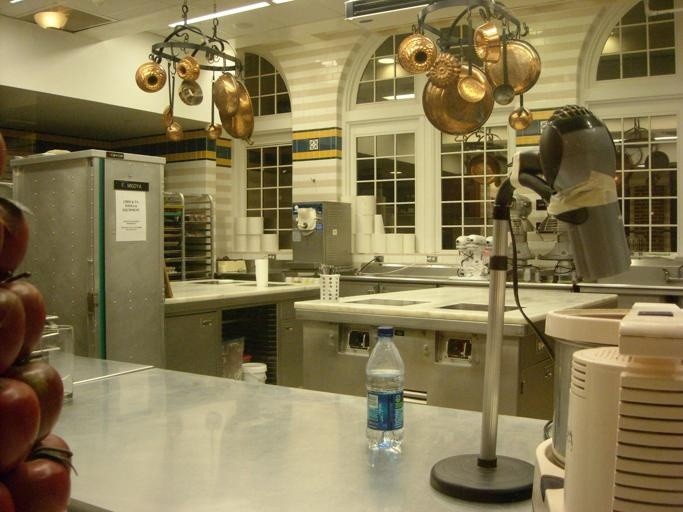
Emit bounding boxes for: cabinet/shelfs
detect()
[163,191,216,281]
[164,289,320,389]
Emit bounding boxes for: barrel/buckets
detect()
[242,363,269,382]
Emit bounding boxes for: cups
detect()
[39,324,75,401]
[255,259,269,288]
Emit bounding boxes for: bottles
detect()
[364,324,405,451]
